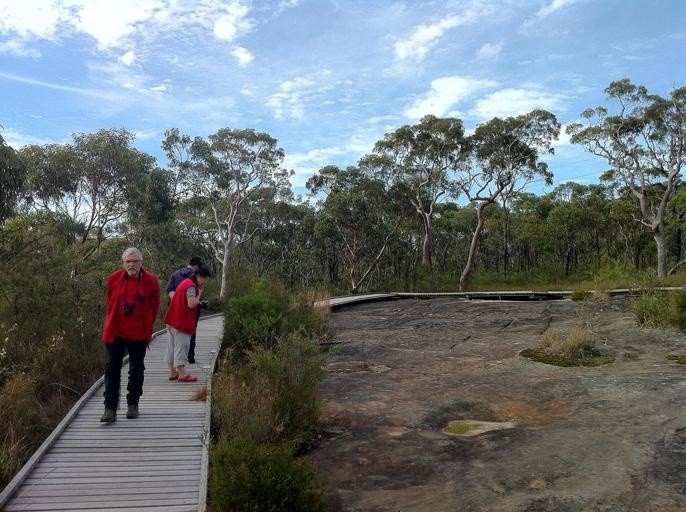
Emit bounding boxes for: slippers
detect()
[169,372,198,382]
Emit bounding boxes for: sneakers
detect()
[101,409,116,422]
[188,357,195,363]
[127,405,138,419]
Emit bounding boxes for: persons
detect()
[165,255,201,364]
[99,247,159,422]
[163,268,212,382]
